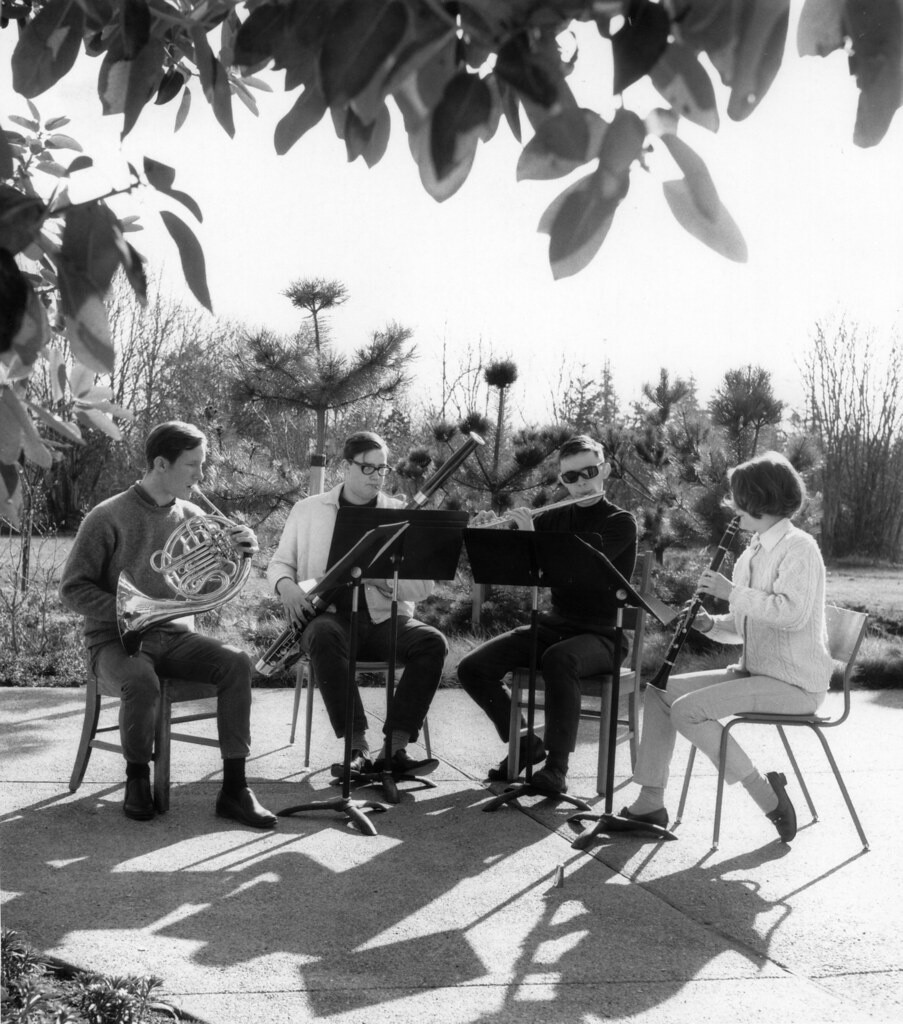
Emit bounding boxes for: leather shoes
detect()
[125,776,155,822]
[373,749,440,782]
[331,749,374,778]
[215,786,279,827]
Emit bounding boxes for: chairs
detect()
[505,550,656,795]
[67,654,226,814]
[672,599,872,853]
[288,641,435,769]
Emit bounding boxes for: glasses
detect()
[559,461,605,484]
[347,458,392,477]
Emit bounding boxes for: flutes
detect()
[646,516,740,693]
[255,431,485,676]
[467,490,605,527]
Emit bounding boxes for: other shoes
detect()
[488,736,548,781]
[532,765,569,793]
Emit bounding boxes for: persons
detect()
[454,432,639,799]
[265,428,450,789]
[56,418,281,827]
[597,446,834,842]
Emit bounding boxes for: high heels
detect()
[764,771,797,842]
[605,805,669,832]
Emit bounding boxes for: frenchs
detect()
[115,483,252,657]
